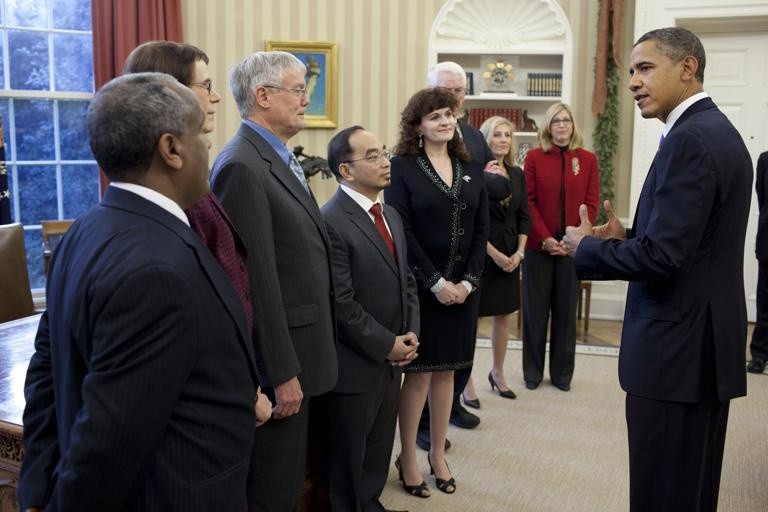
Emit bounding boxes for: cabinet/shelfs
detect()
[463,92,561,138]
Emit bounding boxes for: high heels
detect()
[395,458,431,497]
[459,368,481,409]
[488,368,518,399]
[428,452,456,494]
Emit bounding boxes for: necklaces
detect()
[428,156,449,180]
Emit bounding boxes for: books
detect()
[527,72,562,97]
[464,107,522,131]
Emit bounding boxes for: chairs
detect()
[41,218,80,277]
[0,217,35,321]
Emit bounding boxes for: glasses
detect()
[343,152,390,163]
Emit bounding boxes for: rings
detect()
[495,165,499,170]
[496,170,499,175]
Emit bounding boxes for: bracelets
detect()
[540,240,546,251]
[516,251,525,259]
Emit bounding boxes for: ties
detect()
[288,151,311,198]
[370,204,395,256]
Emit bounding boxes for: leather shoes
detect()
[525,373,572,390]
[451,401,480,428]
[746,357,765,374]
[416,420,451,453]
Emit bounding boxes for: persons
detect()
[319,125,426,511]
[517,103,600,392]
[212,49,339,510]
[746,145,767,376]
[464,116,532,410]
[19,73,260,508]
[385,88,491,497]
[126,42,274,512]
[415,61,513,455]
[558,28,754,511]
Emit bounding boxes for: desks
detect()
[1,307,52,511]
[515,274,590,333]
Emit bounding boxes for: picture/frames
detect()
[259,39,342,129]
